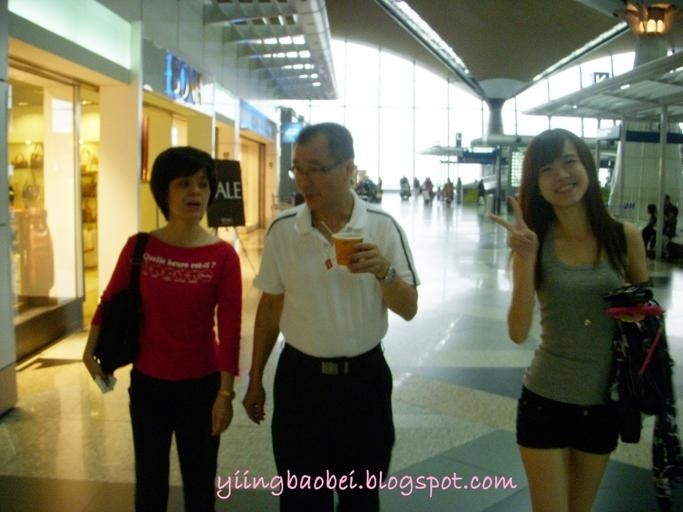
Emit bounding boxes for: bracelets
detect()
[374,260,395,287]
[217,390,236,399]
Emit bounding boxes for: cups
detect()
[330,233,366,267]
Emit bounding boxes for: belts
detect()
[282,343,382,375]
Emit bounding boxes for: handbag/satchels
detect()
[94,231,149,376]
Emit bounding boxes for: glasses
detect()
[290,152,347,179]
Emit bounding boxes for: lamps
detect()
[638,2,667,38]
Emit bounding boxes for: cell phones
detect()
[602,285,640,301]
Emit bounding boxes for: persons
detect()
[79,145,243,512]
[350,175,484,207]
[242,121,420,512]
[641,202,658,258]
[485,128,648,512]
[659,192,679,259]
